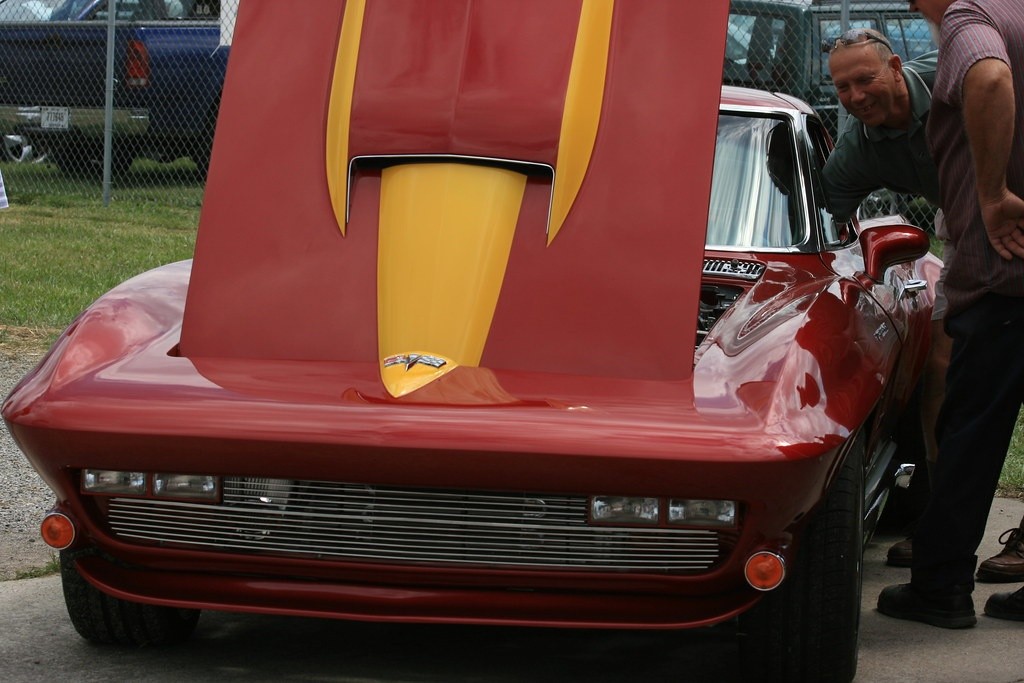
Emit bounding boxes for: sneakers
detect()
[977,517,1024,579]
[877,583,977,628]
[984,586,1024,622]
[887,537,912,566]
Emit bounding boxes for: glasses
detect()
[819,30,894,55]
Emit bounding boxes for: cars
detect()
[2,0,949,683]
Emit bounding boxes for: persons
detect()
[766,123,805,245]
[878,0,1024,629]
[819,26,1024,583]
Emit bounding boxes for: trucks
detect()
[0,0,235,186]
